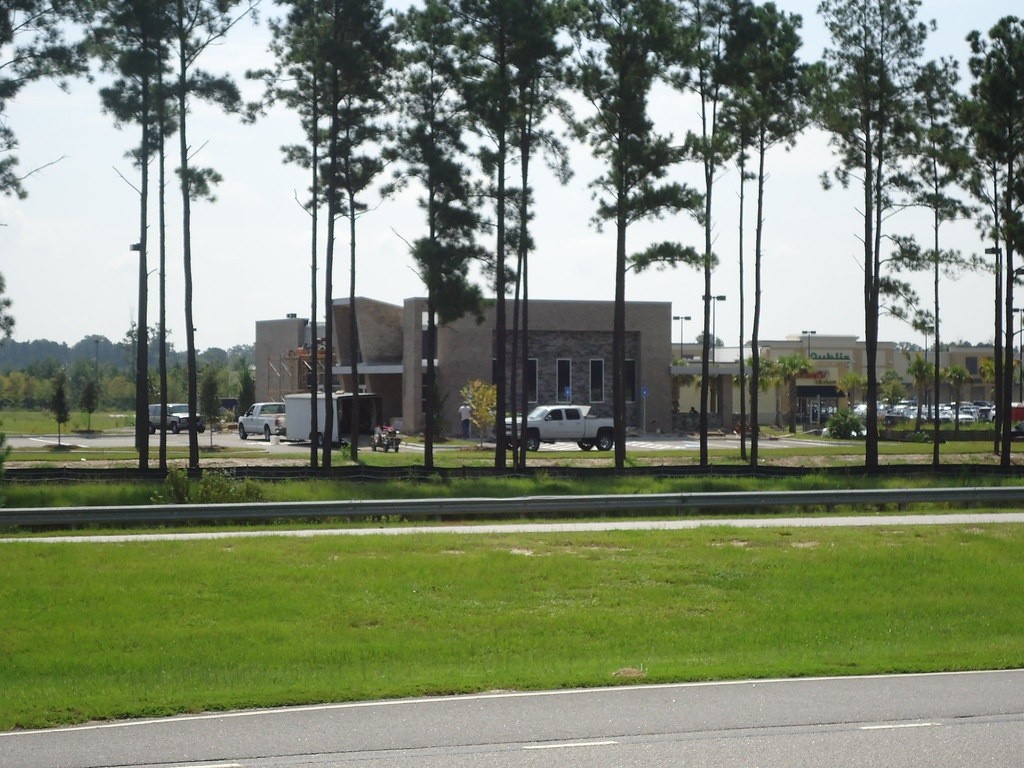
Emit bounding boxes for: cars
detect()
[816,399,1024,425]
[147,403,205,434]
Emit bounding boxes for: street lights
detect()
[985,247,1004,336]
[703,295,727,363]
[673,315,691,364]
[802,330,817,361]
[1013,308,1024,402]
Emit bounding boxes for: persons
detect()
[459,402,471,440]
[689,407,697,416]
[812,402,818,419]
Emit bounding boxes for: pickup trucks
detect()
[236,402,287,443]
[505,404,615,452]
